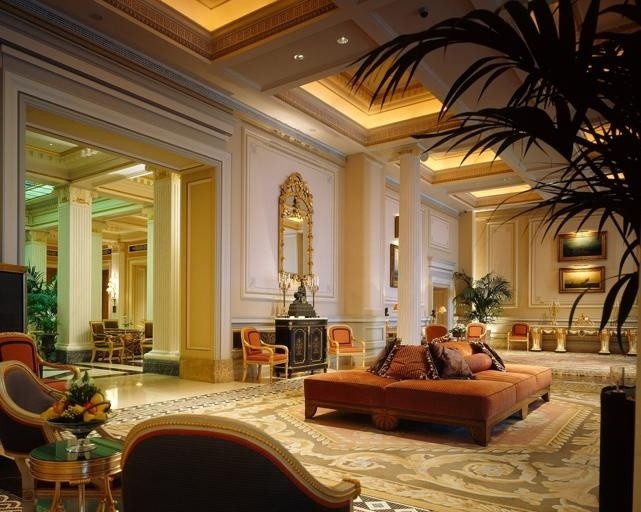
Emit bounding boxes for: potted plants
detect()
[340,0,641,512]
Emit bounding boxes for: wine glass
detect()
[48,417,105,452]
[610,366,626,394]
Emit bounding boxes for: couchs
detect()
[303,365,553,448]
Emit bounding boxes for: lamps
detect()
[432,306,447,317]
[106,272,117,298]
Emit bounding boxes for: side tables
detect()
[30,437,123,512]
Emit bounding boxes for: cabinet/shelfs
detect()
[276,318,328,378]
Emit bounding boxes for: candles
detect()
[278,271,291,285]
[310,275,319,287]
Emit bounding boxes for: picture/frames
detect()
[556,230,607,262]
[559,266,605,293]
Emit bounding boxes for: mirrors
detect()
[276,172,312,280]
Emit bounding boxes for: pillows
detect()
[372,335,505,381]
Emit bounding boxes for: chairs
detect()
[508,323,530,352]
[0,359,112,512]
[467,324,489,342]
[0,330,80,393]
[240,327,289,383]
[89,318,153,363]
[121,414,361,512]
[421,325,447,345]
[328,324,364,372]
